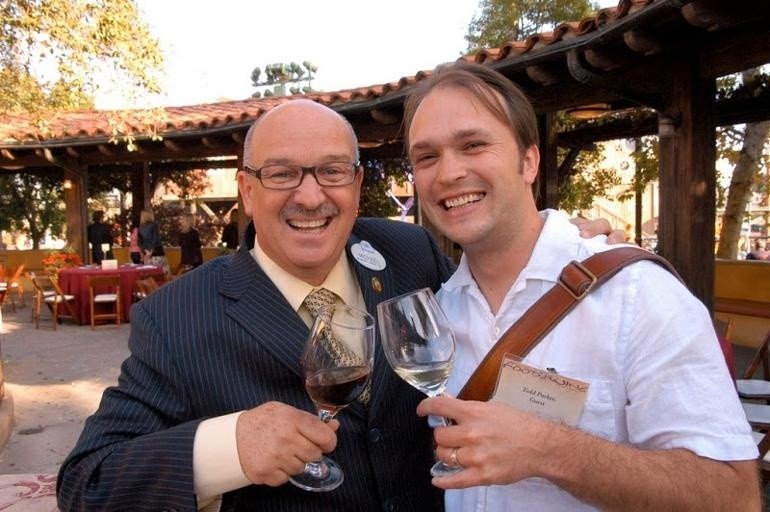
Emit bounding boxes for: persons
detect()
[88,209,116,263]
[181,212,203,267]
[220,205,239,249]
[54,98,636,512]
[129,214,142,264]
[404,60,765,511]
[140,207,170,276]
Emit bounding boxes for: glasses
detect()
[243,160,360,190]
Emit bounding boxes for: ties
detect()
[303,290,372,407]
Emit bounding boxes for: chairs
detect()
[733,329,768,468]
[1,253,172,329]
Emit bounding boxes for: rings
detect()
[449,446,467,470]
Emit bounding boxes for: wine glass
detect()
[286,303,377,490]
[378,288,466,477]
